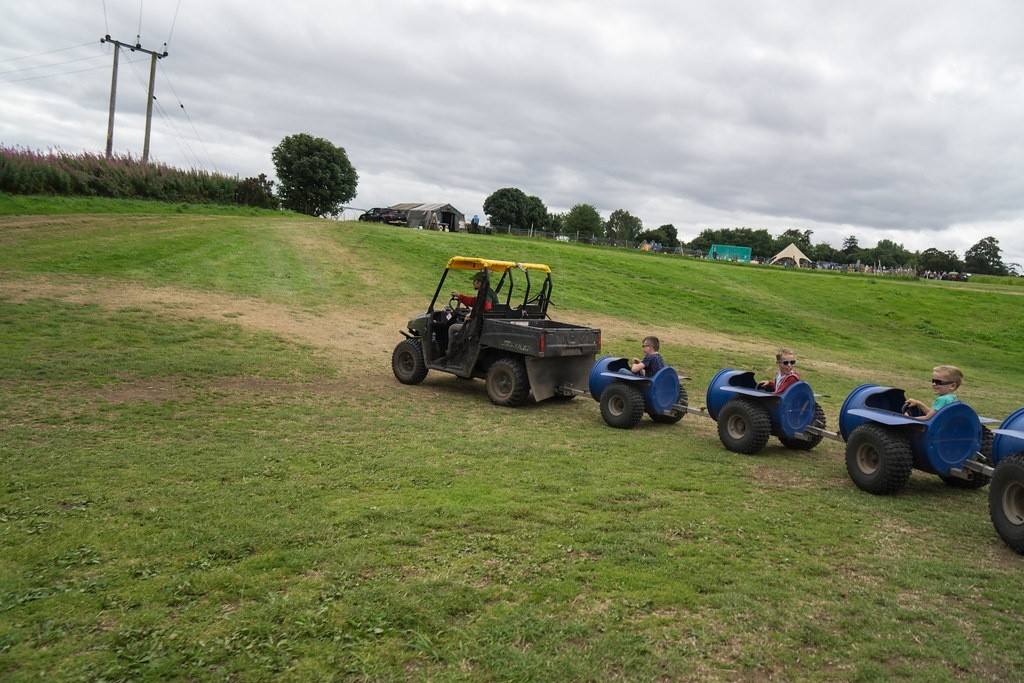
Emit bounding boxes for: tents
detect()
[388,203,466,231]
[768,243,813,268]
[708,245,752,263]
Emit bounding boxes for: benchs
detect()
[483,303,540,318]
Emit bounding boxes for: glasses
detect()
[777,361,795,365]
[642,345,654,348]
[932,378,959,388]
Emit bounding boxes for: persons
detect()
[773,260,954,281]
[471,215,479,234]
[631,335,665,384]
[759,347,800,395]
[447,271,500,350]
[904,364,964,421]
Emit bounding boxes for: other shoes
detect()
[432,357,451,365]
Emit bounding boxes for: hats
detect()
[469,272,490,281]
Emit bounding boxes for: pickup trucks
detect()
[358,207,408,226]
